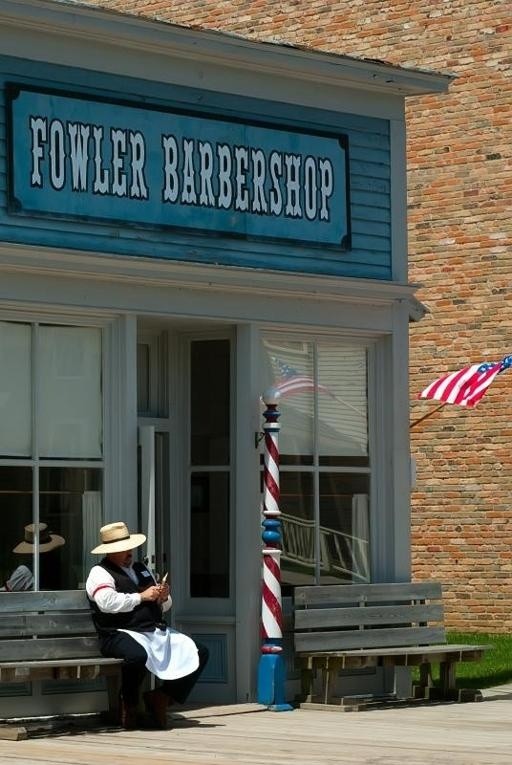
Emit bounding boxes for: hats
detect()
[13,523,65,553]
[90,522,147,554]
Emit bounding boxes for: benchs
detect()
[294,582,490,709]
[0,589,162,740]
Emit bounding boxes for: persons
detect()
[0,523,68,591]
[85,522,210,730]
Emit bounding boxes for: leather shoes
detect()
[145,689,169,729]
[119,689,132,728]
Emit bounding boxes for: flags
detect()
[416,355,512,410]
[258,351,327,410]
